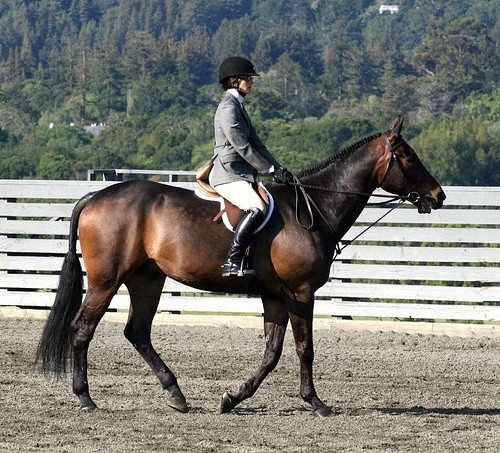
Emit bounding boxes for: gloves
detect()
[271,167,293,184]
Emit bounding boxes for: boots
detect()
[219,207,256,278]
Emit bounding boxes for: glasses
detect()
[237,76,253,81]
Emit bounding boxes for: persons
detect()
[208,56,293,276]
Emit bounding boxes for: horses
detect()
[34,114,446,419]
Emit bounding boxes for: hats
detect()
[218,57,259,82]
